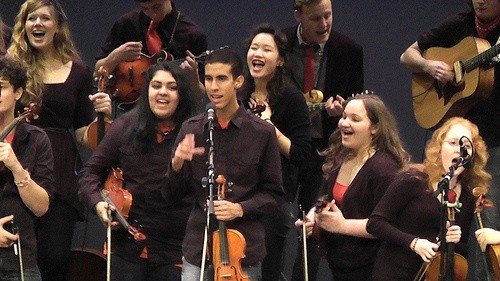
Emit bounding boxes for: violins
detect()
[473,186,500,281]
[413,190,468,281]
[207,175,249,281]
[290,197,329,281]
[0,102,39,169]
[249,100,266,113]
[100,167,146,241]
[74,66,112,164]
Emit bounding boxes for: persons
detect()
[94,0,213,96]
[68,58,198,281]
[0,54,52,281]
[162,50,284,281]
[366,117,492,281]
[475,227,500,252]
[294,94,413,281]
[276,0,368,218]
[401,0,500,145]
[235,24,314,281]
[5,0,113,281]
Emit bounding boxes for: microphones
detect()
[205,102,216,128]
[458,140,471,169]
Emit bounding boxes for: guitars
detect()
[305,90,374,138]
[411,36,500,129]
[107,45,230,106]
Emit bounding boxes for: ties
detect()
[446,179,459,203]
[303,45,319,92]
[145,20,162,56]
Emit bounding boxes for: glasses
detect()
[441,138,473,149]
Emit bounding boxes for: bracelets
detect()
[412,238,420,253]
[265,118,271,121]
[14,169,31,188]
